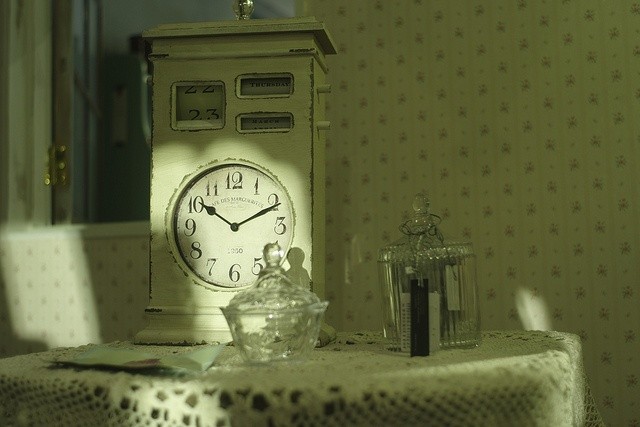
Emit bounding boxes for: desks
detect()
[0,326,607,425]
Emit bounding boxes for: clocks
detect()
[130,1,337,348]
